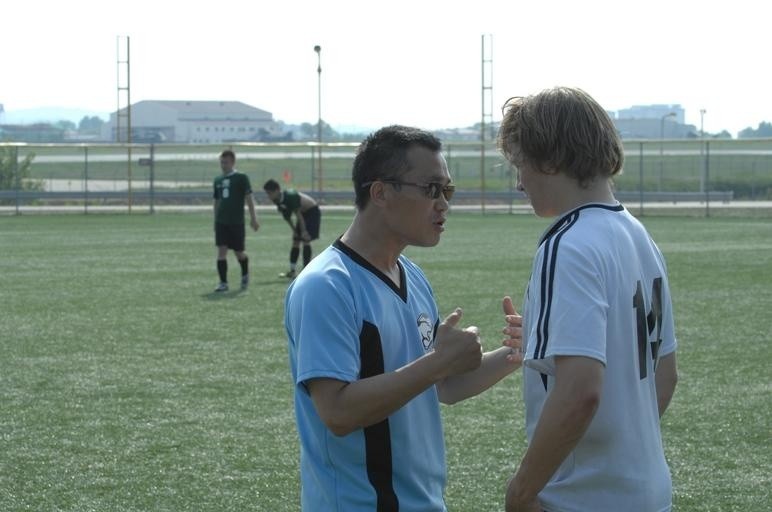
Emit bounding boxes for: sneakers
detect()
[213,270,296,292]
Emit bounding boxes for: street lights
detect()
[700,109,706,194]
[660,112,677,191]
[314,44,322,193]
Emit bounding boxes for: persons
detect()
[213,150,322,292]
[496,89,675,510]
[285,126,524,511]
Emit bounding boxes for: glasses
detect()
[358,179,456,201]
